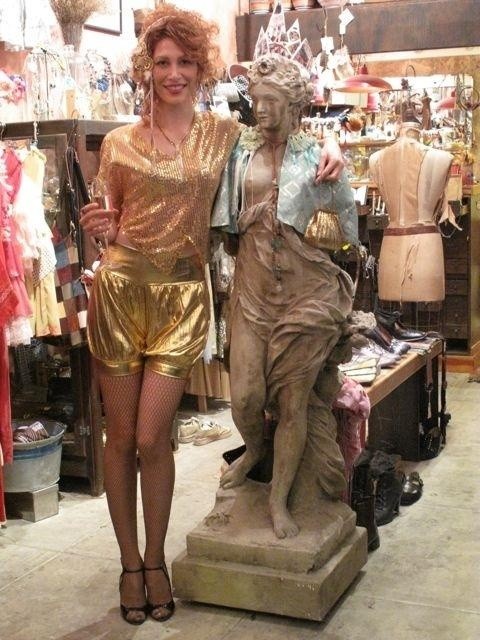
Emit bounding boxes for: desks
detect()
[277,335,451,517]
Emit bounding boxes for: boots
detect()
[351,463,406,553]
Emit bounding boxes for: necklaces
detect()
[155,114,195,153]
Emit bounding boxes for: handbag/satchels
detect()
[304,209,344,251]
[32,221,90,349]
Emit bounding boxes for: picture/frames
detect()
[82,0,123,39]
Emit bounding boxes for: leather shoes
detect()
[420,427,443,460]
[352,310,426,368]
[400,472,424,506]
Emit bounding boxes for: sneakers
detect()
[179,416,232,446]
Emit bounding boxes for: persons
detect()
[79,0,343,625]
[368,122,454,303]
[219,54,359,539]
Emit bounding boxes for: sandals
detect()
[119,563,147,625]
[144,561,175,622]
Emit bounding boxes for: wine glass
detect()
[84,180,126,272]
